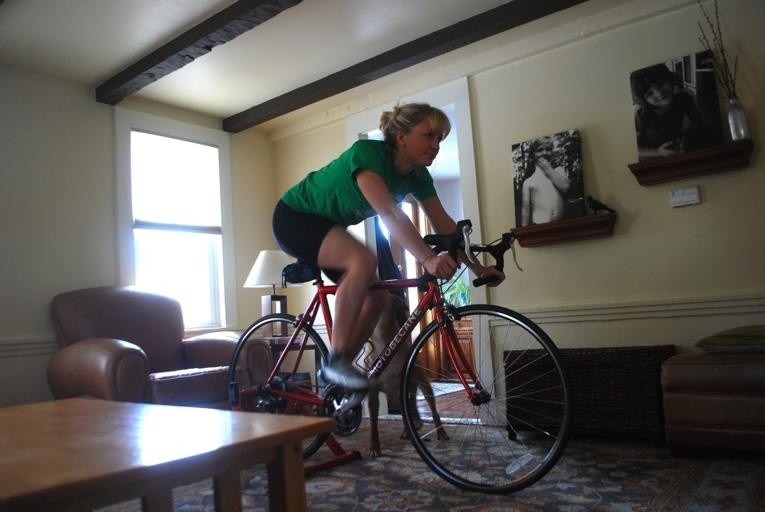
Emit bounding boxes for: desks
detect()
[1,396,337,512]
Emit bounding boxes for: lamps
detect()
[242,250,307,338]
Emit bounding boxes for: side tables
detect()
[269,343,322,395]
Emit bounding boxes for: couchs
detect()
[660,354,764,459]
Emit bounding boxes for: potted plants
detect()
[696,0,751,141]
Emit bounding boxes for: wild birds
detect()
[585,193,616,216]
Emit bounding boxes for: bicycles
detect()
[228,218,574,495]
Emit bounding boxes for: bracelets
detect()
[421,252,436,266]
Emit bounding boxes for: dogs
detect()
[361,284,450,461]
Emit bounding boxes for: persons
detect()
[272,104,506,389]
[521,136,570,228]
[631,62,704,160]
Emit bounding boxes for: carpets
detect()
[415,383,480,418]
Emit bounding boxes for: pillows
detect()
[695,325,765,354]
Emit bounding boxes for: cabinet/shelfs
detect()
[439,315,475,379]
[504,345,676,440]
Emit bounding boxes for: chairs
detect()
[47,286,275,409]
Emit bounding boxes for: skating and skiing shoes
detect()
[319,360,371,391]
